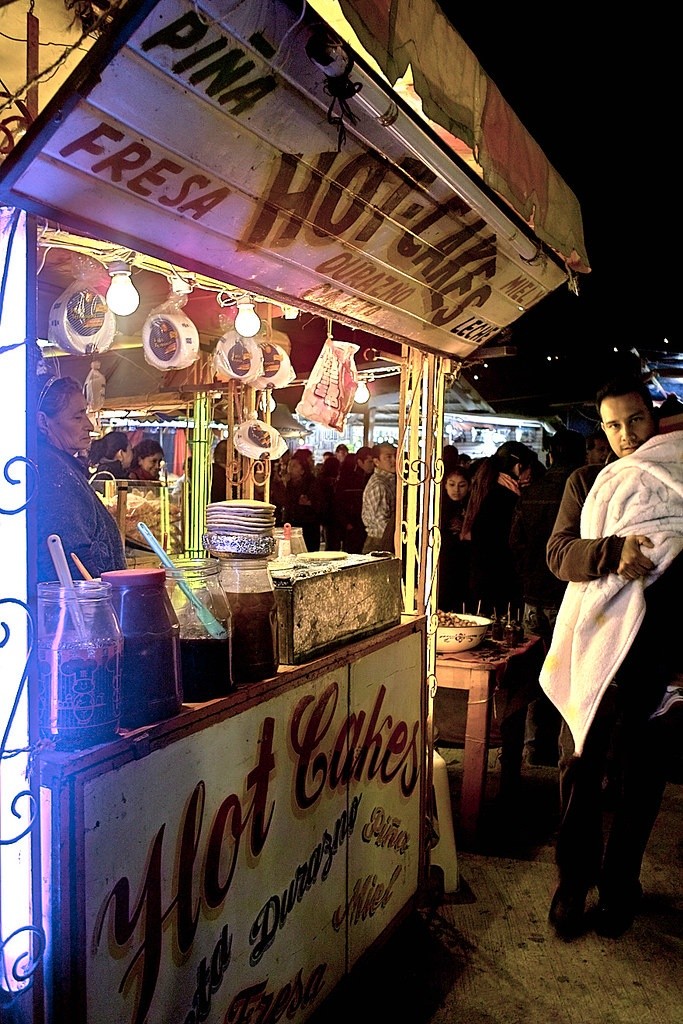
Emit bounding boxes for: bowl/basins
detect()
[436,612,495,653]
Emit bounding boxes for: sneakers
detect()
[595,677,621,729]
[648,688,683,721]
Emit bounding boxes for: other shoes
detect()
[596,881,643,938]
[548,869,601,930]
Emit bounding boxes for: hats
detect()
[656,394,683,435]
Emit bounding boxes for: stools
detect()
[424,746,460,896]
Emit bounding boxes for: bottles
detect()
[37,579,124,752]
[217,557,282,684]
[94,567,185,732]
[159,557,234,704]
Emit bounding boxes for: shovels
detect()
[137,522,228,640]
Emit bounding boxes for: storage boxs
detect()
[274,551,402,668]
[115,479,184,555]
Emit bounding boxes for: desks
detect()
[436,633,548,843]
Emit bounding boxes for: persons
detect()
[536,390,683,757]
[549,379,683,939]
[36,372,128,631]
[438,429,611,764]
[172,456,192,495]
[78,431,164,496]
[210,438,396,554]
